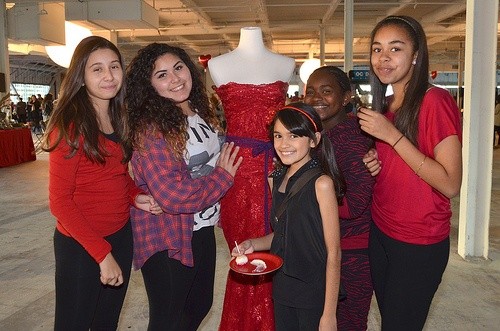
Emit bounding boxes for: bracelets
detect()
[415,155,428,174]
[392,135,405,148]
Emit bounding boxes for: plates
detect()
[229,252,284,276]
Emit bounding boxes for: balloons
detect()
[431,70,438,79]
[199,54,211,68]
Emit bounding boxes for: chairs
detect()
[33,122,48,149]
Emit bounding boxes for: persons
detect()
[11,14,500,331]
[207,28,299,331]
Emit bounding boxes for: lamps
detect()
[0,127,36,168]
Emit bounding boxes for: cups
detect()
[360,94,385,111]
